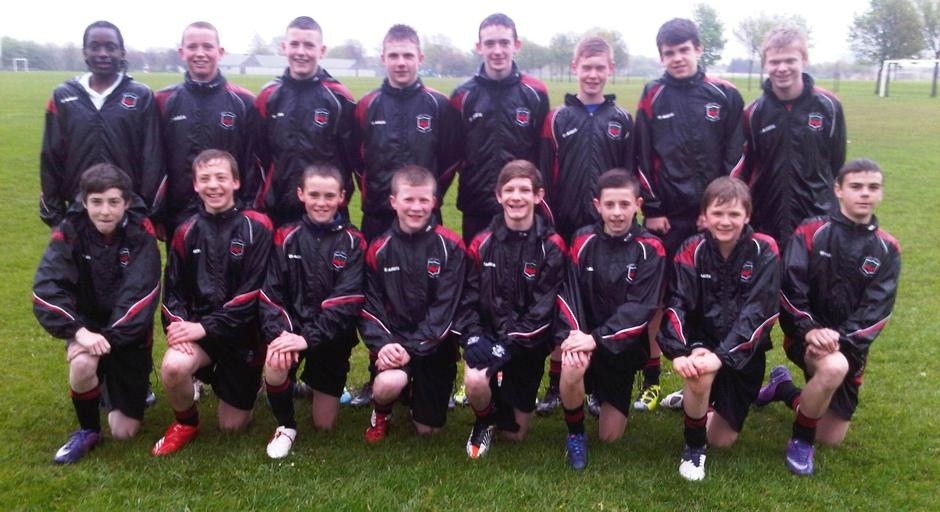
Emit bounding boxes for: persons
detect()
[31,13,901,481]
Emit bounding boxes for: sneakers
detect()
[678,444,708,481]
[448,384,468,408]
[340,382,374,407]
[785,437,815,475]
[145,390,155,406]
[634,384,661,411]
[756,366,792,406]
[193,379,203,401]
[54,428,103,462]
[151,420,202,456]
[364,409,392,442]
[466,423,496,459]
[586,393,601,416]
[660,388,684,408]
[267,426,296,458]
[536,386,561,416]
[567,431,587,469]
